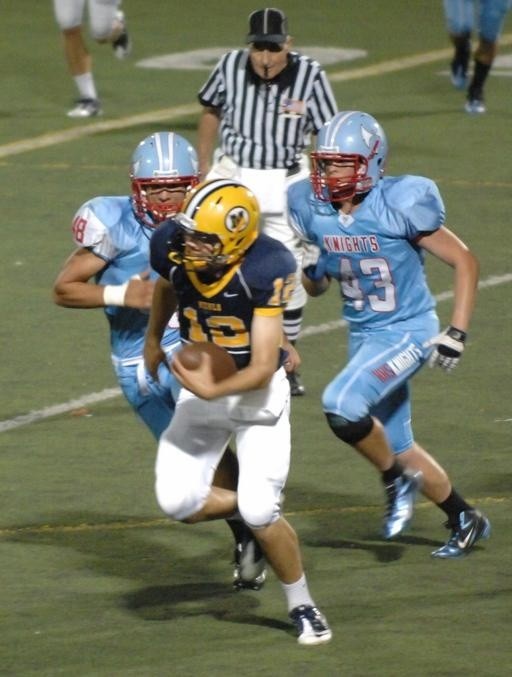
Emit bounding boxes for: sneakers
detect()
[465,82,485,113]
[450,41,470,87]
[431,508,490,559]
[111,11,129,58]
[222,513,268,591]
[285,369,307,398]
[289,603,334,648]
[67,99,104,119]
[381,465,424,544]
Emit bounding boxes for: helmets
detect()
[310,110,387,203]
[129,131,202,219]
[169,180,261,278]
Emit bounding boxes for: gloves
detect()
[421,326,467,373]
[303,244,326,282]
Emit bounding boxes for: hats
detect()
[247,7,287,45]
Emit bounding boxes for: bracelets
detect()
[104,284,126,307]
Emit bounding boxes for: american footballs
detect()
[173,342,236,382]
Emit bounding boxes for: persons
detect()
[138,177,334,647]
[284,109,493,560]
[193,5,341,398]
[53,0,132,119]
[442,0,512,113]
[53,130,305,592]
